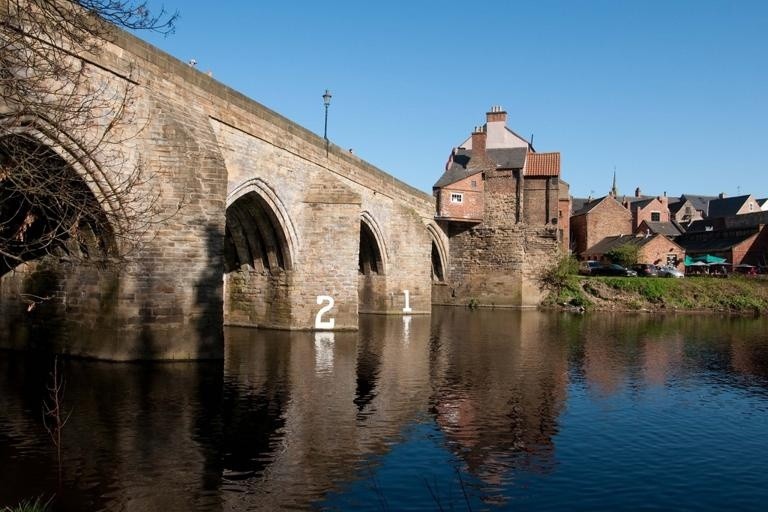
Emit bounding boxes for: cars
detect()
[579,259,684,279]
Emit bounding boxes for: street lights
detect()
[320,88,331,138]
